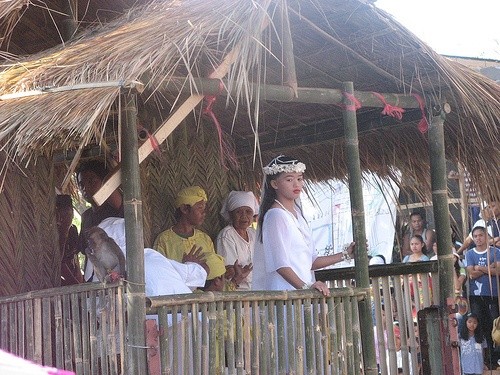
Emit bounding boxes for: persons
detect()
[54,154,500,375]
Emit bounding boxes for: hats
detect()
[175,185,208,208]
[219,190,260,221]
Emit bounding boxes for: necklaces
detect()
[274,199,312,240]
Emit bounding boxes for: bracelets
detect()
[232,276,240,287]
[302,283,310,290]
[339,243,352,264]
